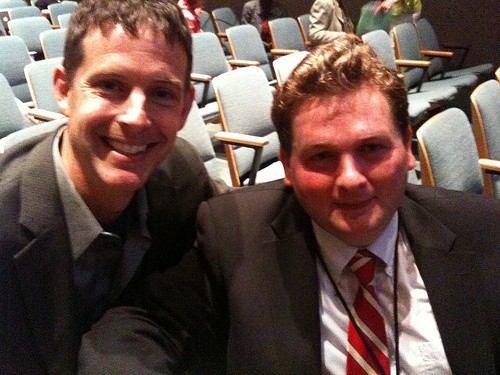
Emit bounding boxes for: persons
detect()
[0,0,247,375]
[78,31,499,375]
[176,0,422,50]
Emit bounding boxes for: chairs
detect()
[0,0,500,202]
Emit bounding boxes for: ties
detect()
[343,247,393,374]
